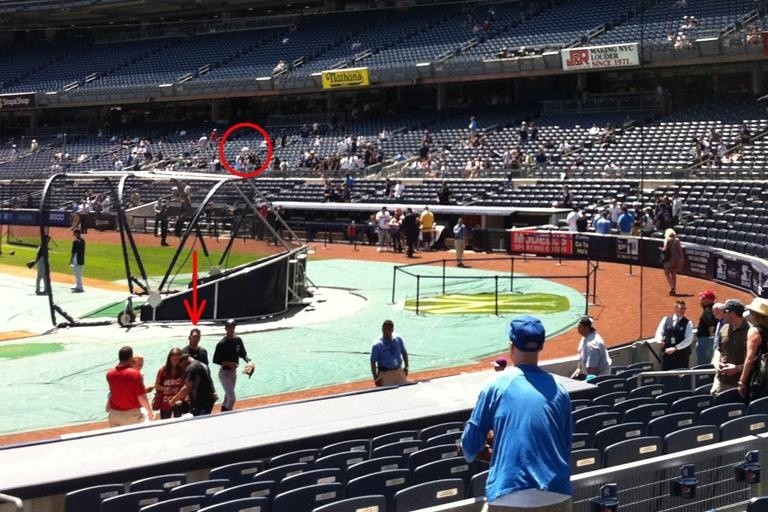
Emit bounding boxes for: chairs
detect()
[359,0,768,273]
[0,2,360,231]
[62,353,768,511]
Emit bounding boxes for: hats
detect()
[700,290,715,299]
[718,299,744,313]
[509,315,545,351]
[580,316,596,331]
[745,298,768,316]
[490,359,506,368]
[225,318,237,326]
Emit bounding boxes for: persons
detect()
[460,313,575,511]
[2,13,767,426]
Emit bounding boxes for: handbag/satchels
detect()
[153,392,162,410]
[661,247,671,263]
